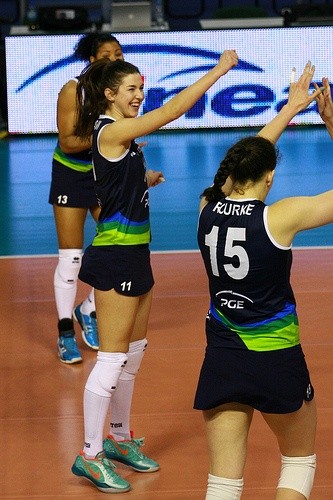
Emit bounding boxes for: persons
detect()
[48,33,164,364]
[194,60,333,500]
[71,50,240,493]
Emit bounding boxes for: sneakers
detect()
[103,430,160,472]
[72,302,99,350]
[57,331,83,364]
[70,450,130,493]
